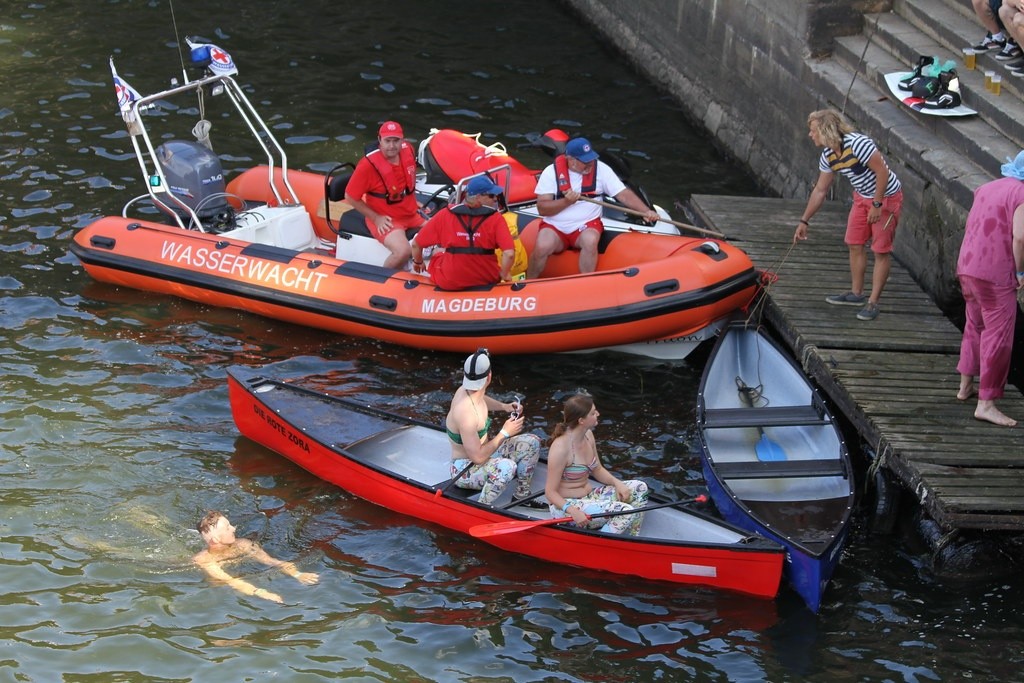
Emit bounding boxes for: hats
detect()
[463,353,490,391]
[566,138,599,163]
[1001,150,1024,180]
[466,176,503,196]
[379,121,403,139]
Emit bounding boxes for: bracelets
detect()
[500,429,509,439]
[800,219,809,226]
[412,258,424,264]
[1016,271,1024,277]
[562,502,572,513]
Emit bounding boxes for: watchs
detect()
[872,200,882,208]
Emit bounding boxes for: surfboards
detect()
[885,70,978,117]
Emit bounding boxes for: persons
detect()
[545,394,648,537]
[344,121,429,270]
[446,349,549,510]
[971,0,1024,77]
[526,138,659,280]
[956,149,1024,427]
[793,108,904,320]
[412,174,515,291]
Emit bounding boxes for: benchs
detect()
[702,405,829,428]
[431,478,482,498]
[714,459,847,480]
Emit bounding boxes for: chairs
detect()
[324,163,375,239]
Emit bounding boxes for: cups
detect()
[985,71,995,89]
[991,75,1002,95]
[963,47,973,65]
[965,50,976,69]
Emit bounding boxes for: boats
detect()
[416,158,681,255]
[694,312,858,613]
[66,0,780,355]
[235,372,787,602]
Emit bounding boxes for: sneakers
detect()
[857,303,879,321]
[971,31,1006,53]
[826,291,865,306]
[995,37,1024,60]
[925,68,962,109]
[898,55,934,91]
[1004,59,1024,77]
[511,493,549,512]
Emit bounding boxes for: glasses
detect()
[511,396,520,422]
[479,193,495,199]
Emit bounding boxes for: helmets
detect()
[912,76,939,99]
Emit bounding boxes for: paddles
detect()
[738,387,789,461]
[433,461,474,501]
[469,491,707,537]
[561,190,727,239]
[316,198,355,221]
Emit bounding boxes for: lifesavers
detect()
[858,464,902,534]
